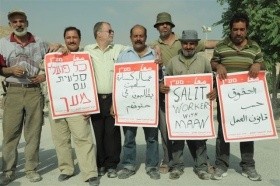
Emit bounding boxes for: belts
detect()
[98,95,112,99]
[5,83,40,88]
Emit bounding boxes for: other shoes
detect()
[98,167,105,176]
[159,163,170,174]
[26,172,41,182]
[206,163,214,174]
[197,169,211,179]
[58,173,75,181]
[118,168,136,179]
[147,168,160,179]
[108,168,117,178]
[89,177,99,186]
[0,174,15,185]
[169,167,183,179]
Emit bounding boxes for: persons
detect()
[149,12,222,174]
[110,24,162,180]
[38,26,100,186]
[210,14,267,180]
[159,30,217,179]
[46,21,129,177]
[0,11,48,185]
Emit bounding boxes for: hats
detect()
[7,9,26,21]
[153,12,175,28]
[178,30,202,41]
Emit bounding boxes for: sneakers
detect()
[242,166,261,181]
[212,168,228,180]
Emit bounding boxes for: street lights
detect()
[201,25,212,40]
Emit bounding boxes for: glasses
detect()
[99,29,114,34]
